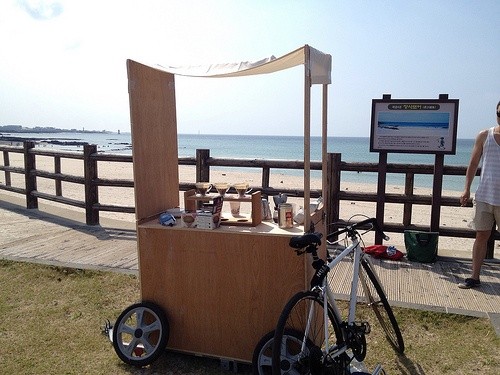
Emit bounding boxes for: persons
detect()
[457,101,500,288]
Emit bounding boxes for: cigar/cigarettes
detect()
[460,203,466,206]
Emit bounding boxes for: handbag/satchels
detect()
[404,230,439,263]
[360,245,404,261]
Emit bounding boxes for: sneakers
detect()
[458,278,481,289]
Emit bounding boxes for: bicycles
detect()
[271,214,405,375]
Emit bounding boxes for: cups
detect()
[195,183,212,196]
[215,182,230,195]
[230,202,240,215]
[233,182,253,197]
[278,204,293,228]
